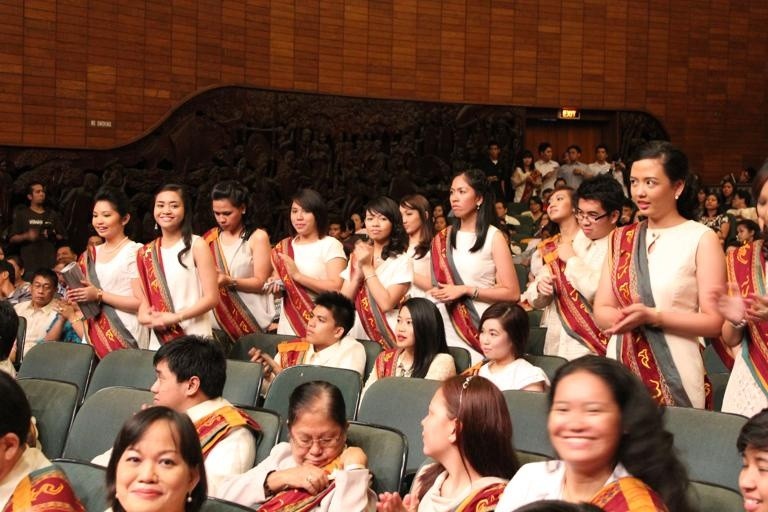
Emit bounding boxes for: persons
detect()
[494,355,698,512]
[737,409,768,512]
[512,499,605,512]
[376,375,520,512]
[92,334,263,498]
[0,371,86,512]
[214,381,378,512]
[105,406,207,512]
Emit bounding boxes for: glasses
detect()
[288,420,348,450]
[574,206,617,224]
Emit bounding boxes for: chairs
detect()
[12,310,750,512]
[514,263,532,294]
